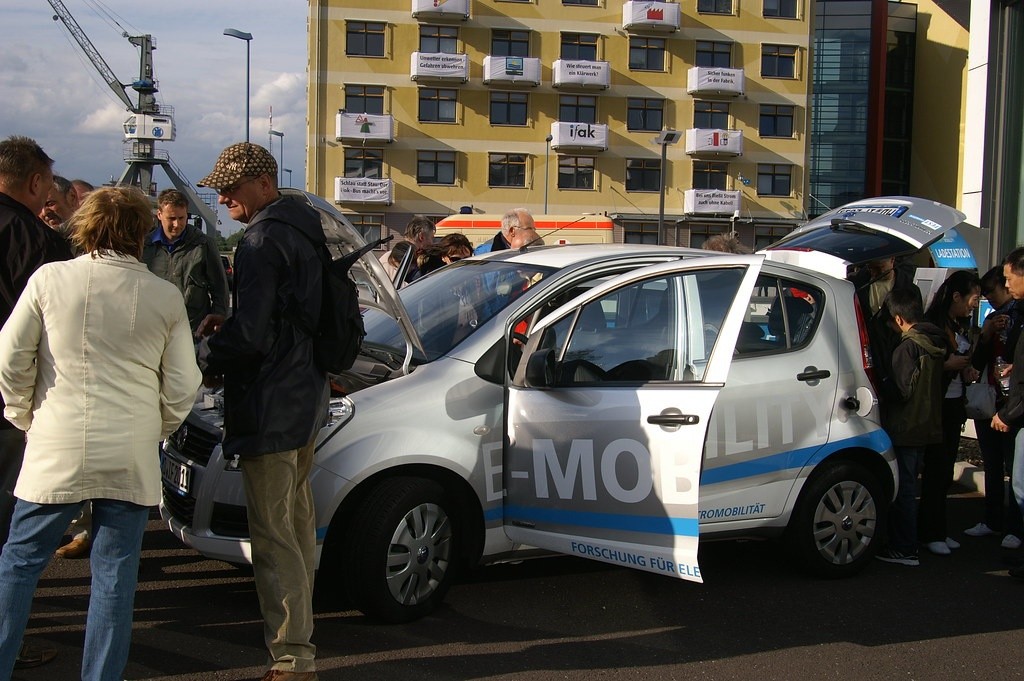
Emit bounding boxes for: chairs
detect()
[746,294,813,352]
[567,285,606,339]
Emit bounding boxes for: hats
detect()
[197,143,278,190]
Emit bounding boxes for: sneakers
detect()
[873,549,920,566]
[55,539,91,558]
[964,522,1001,536]
[922,536,961,555]
[1001,535,1022,549]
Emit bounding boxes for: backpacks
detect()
[311,238,368,376]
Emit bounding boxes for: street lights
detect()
[659,130,684,243]
[545,135,553,215]
[267,129,285,186]
[281,167,292,188]
[223,28,256,143]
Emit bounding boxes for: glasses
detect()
[214,176,259,195]
[446,255,471,262]
[514,226,536,231]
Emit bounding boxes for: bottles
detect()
[994,356,1011,396]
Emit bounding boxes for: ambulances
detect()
[433,204,615,255]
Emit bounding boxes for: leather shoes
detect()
[13,642,57,669]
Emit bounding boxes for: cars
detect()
[154,185,964,626]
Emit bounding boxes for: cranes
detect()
[47,0,217,242]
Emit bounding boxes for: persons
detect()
[199,140,330,681]
[767,244,1023,566]
[0,186,204,681]
[695,233,747,320]
[1,134,230,560]
[372,212,577,346]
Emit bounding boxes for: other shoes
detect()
[1008,567,1024,579]
[260,670,319,681]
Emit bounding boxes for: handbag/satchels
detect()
[961,362,997,419]
